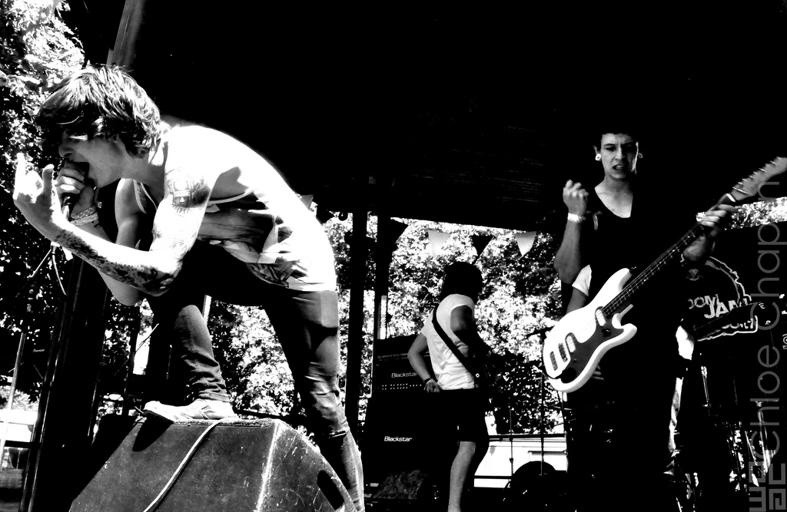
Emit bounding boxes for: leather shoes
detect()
[143,397,242,424]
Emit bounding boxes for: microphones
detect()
[522,327,552,340]
[50,162,88,256]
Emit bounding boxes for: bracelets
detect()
[423,376,432,384]
[71,206,105,226]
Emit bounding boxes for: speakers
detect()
[61,414,357,511]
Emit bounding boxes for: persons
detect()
[13,66,365,511]
[407,262,490,512]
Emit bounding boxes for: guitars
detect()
[544,154,787,394]
[674,292,787,367]
